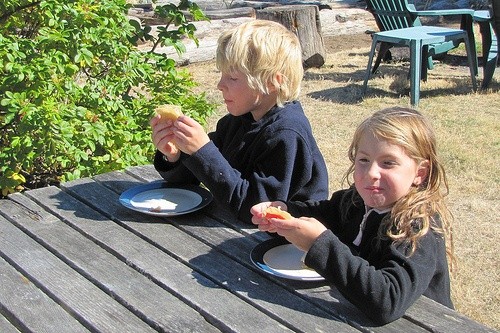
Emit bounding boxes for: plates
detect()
[250,237,325,280]
[119,182,212,217]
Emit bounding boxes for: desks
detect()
[0,163,493,332]
[473,11,499,91]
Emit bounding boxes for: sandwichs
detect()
[156,105,184,150]
[263,206,294,222]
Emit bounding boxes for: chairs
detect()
[366,0,477,87]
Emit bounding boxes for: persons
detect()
[149,20,329,225]
[250,105,454,325]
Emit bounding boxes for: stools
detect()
[358,25,479,107]
